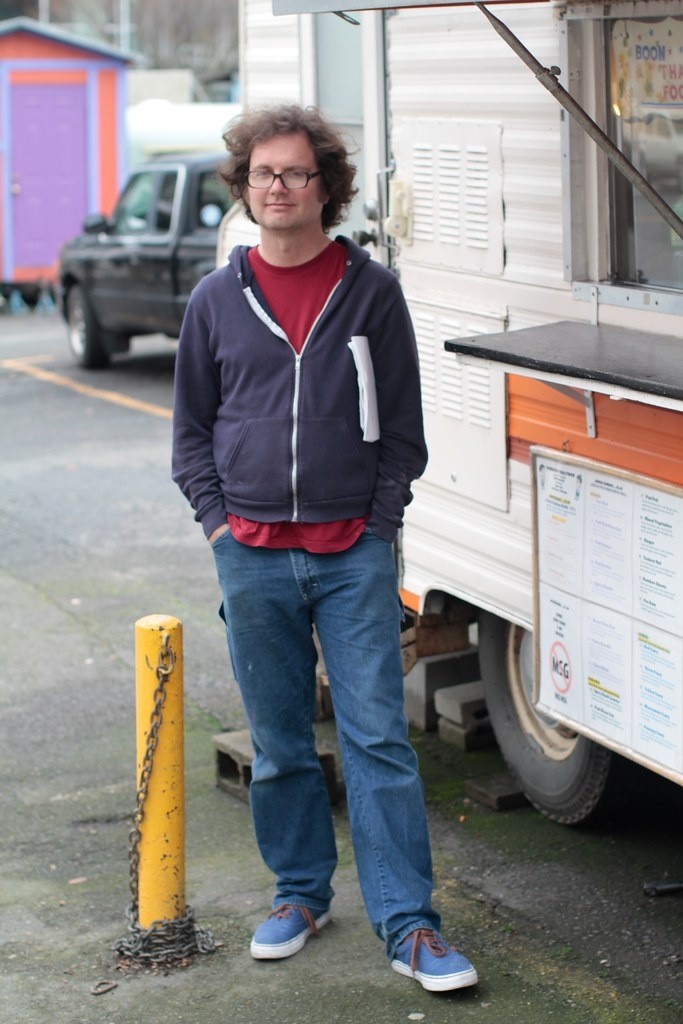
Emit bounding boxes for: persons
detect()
[172,104,479,994]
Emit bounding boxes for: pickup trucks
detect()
[55,151,234,372]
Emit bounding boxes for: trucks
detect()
[237,0,682,826]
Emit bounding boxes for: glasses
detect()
[242,170,322,188]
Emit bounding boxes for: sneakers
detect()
[391,927,477,991]
[248,904,332,958]
